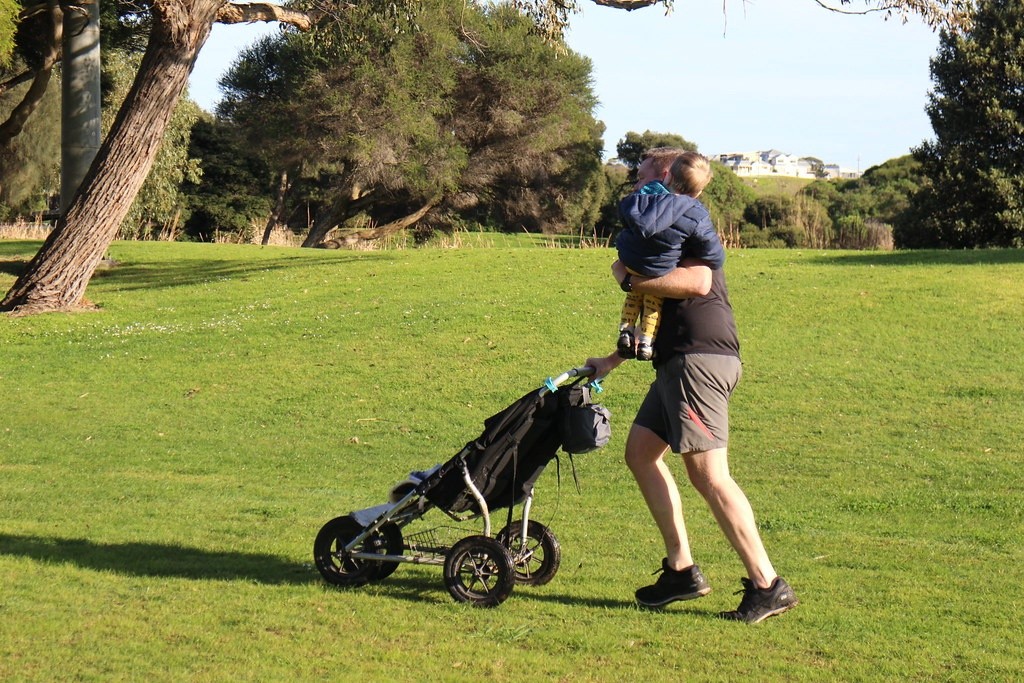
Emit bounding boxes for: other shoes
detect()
[637,343,659,361]
[617,330,636,359]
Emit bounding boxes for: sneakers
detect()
[716,575,799,628]
[635,558,711,608]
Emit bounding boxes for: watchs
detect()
[621,273,632,292]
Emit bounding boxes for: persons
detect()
[617,153,724,361]
[584,145,799,625]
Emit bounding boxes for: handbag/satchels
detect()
[562,388,612,454]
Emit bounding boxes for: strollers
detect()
[311,364,595,613]
